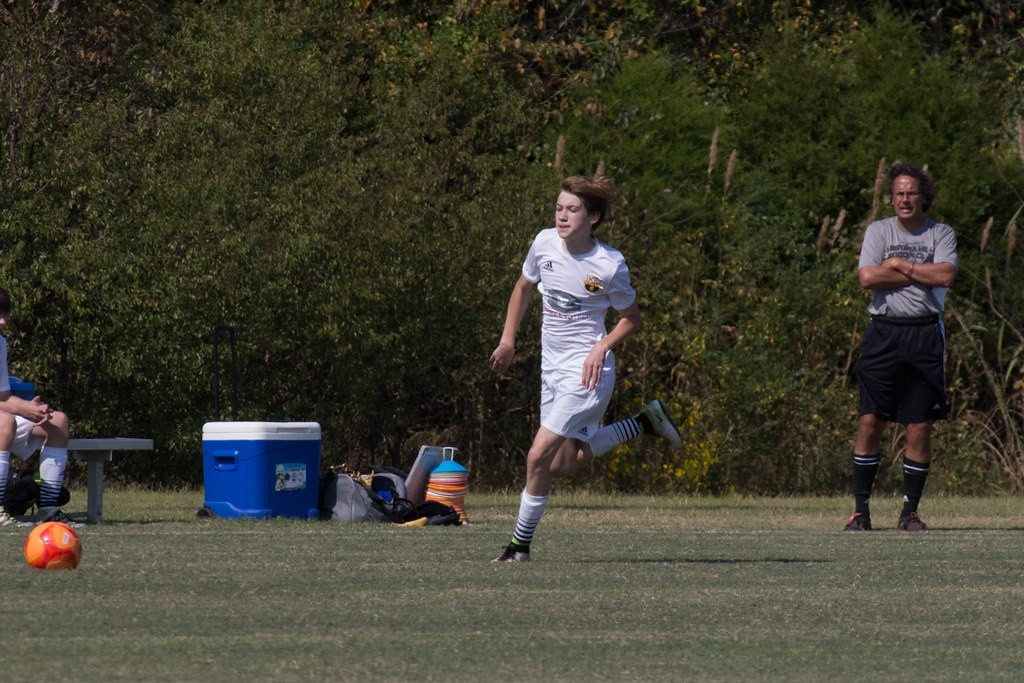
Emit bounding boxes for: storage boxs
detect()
[198,421,322,519]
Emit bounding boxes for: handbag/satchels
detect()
[328,466,462,524]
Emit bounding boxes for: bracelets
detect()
[908,263,914,276]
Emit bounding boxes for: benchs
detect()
[37,438,154,522]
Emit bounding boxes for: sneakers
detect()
[491,543,533,564]
[895,516,928,530]
[0,507,40,533]
[33,509,87,529]
[843,515,873,530]
[639,399,684,451]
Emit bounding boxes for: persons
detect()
[489,178,684,563]
[0,287,85,530]
[843,164,959,531]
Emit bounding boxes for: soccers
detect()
[24,522,83,571]
